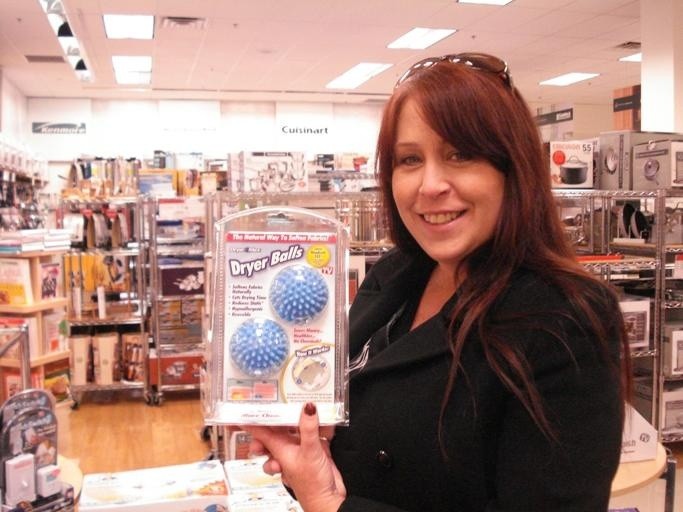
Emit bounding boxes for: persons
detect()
[226,52,624,512]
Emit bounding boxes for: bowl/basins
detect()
[268,149,305,191]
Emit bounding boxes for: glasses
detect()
[392,52,514,92]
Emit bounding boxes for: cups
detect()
[249,168,277,195]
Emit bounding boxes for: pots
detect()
[600,150,619,180]
[615,201,650,238]
[559,155,588,185]
[642,158,661,186]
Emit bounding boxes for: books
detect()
[0,228,74,252]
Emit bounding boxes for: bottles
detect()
[335,191,390,241]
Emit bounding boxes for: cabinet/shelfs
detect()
[55,192,208,401]
[553,189,682,443]
[4,249,70,405]
